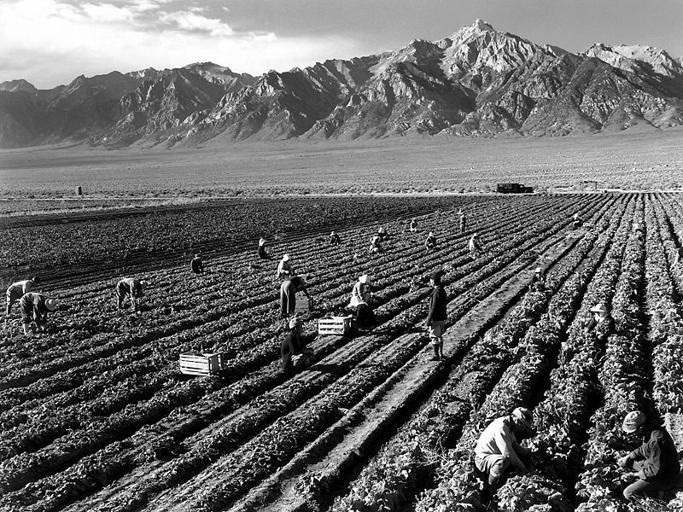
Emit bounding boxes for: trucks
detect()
[497,184,533,193]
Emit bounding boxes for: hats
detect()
[621,410,647,434]
[288,316,303,330]
[358,274,371,285]
[511,406,533,432]
[283,254,291,262]
[347,295,364,308]
[45,298,56,311]
[258,237,267,246]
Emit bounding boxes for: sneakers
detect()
[428,355,448,361]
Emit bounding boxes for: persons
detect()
[115,278,146,308]
[6,276,40,314]
[190,253,203,273]
[616,410,679,501]
[19,292,56,334]
[529,266,544,292]
[258,238,270,258]
[572,214,583,230]
[474,407,537,486]
[278,212,482,370]
